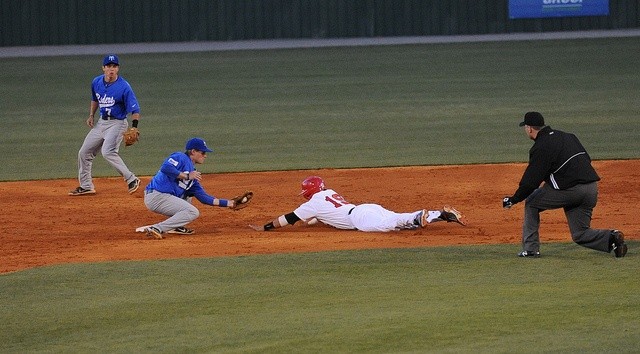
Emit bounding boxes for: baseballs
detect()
[240,197,248,204]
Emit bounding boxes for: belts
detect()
[348,207,355,215]
[102,116,114,120]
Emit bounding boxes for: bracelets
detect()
[90,114,94,116]
[132,119,139,128]
[263,220,275,231]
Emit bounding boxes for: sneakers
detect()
[146,226,162,239]
[503,196,513,209]
[128,177,141,194]
[67,186,96,196]
[517,250,542,257]
[610,229,627,257]
[166,226,195,235]
[443,205,468,226]
[416,209,429,227]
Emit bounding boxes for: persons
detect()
[66,53,142,198]
[143,137,255,241]
[248,174,467,234]
[501,110,628,259]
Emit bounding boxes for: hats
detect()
[520,112,545,126]
[103,54,119,66]
[185,138,214,152]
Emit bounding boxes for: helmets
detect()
[297,176,327,201]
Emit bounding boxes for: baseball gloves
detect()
[123,127,140,146]
[232,192,253,210]
